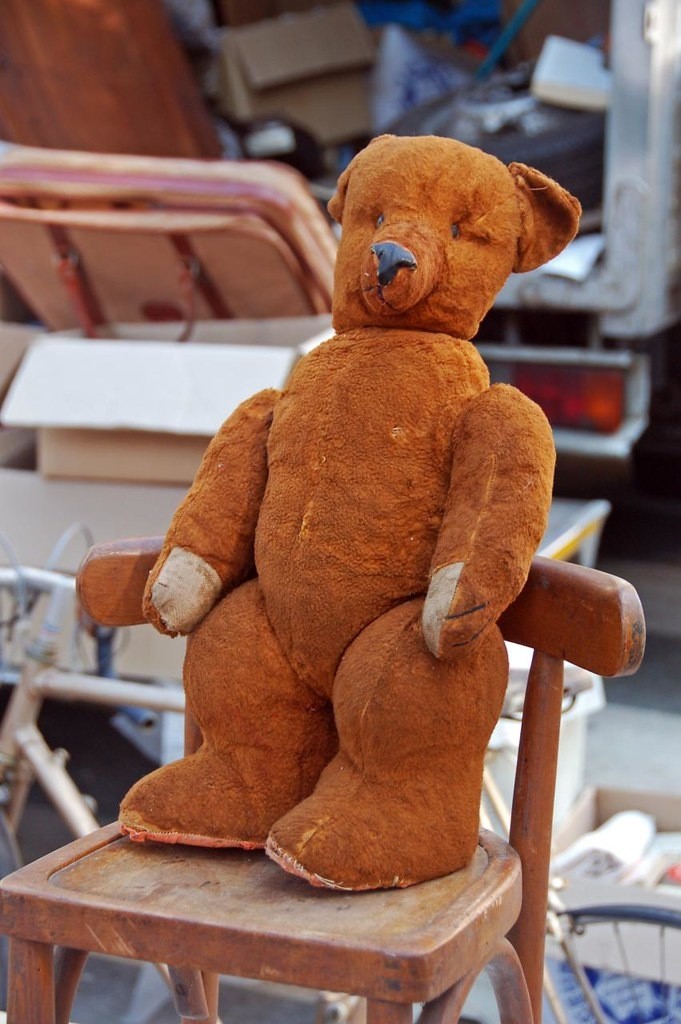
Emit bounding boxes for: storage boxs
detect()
[551,784,681,987]
[0,318,46,466]
[1,313,337,484]
[199,2,375,144]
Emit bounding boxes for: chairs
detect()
[0,534,646,1024]
[0,139,339,333]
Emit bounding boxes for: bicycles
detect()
[0,518,681,1024]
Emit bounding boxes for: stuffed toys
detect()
[119,132,582,892]
